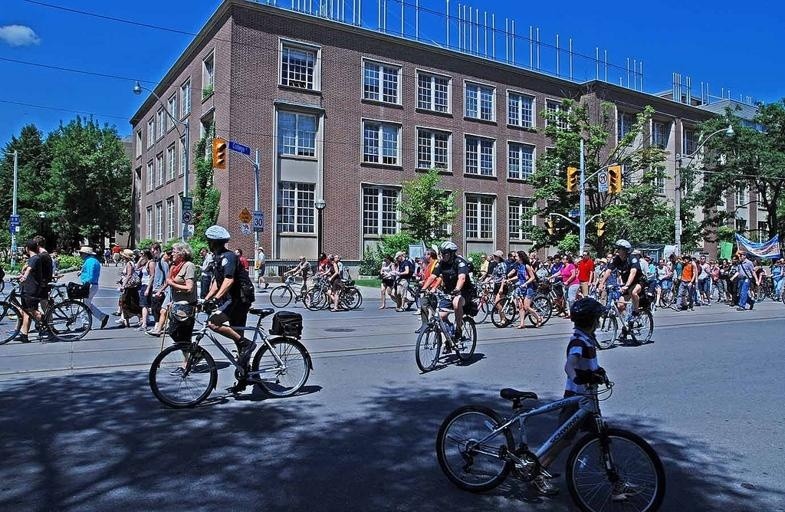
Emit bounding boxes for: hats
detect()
[77,247,96,255]
[394,251,403,259]
[493,250,504,260]
[120,249,136,258]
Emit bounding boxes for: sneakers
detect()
[238,340,256,359]
[611,482,646,500]
[8,328,58,342]
[171,367,185,375]
[101,315,160,337]
[533,475,559,495]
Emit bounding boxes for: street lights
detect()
[315,198,327,269]
[38,212,45,237]
[133,80,189,245]
[755,185,784,241]
[676,125,735,255]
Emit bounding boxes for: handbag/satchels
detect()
[128,273,141,287]
[205,225,230,242]
[169,300,191,322]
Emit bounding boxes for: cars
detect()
[7,247,27,264]
[0,247,8,263]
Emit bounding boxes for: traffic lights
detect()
[597,220,606,237]
[567,167,578,192]
[212,138,227,170]
[608,166,621,193]
[544,219,554,237]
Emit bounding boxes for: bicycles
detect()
[302,276,363,312]
[592,284,654,350]
[436,366,666,512]
[0,276,92,345]
[415,290,477,372]
[149,297,313,409]
[0,266,4,292]
[471,269,785,328]
[270,274,311,309]
[3,268,79,321]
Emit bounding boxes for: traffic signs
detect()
[253,210,265,232]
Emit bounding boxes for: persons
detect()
[94,242,123,267]
[110,242,214,337]
[525,296,647,500]
[234,249,249,276]
[1,235,109,343]
[194,225,260,393]
[256,246,269,289]
[378,239,785,355]
[284,252,352,312]
[166,242,197,376]
[735,233,781,260]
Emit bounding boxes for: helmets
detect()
[616,239,632,249]
[570,298,607,320]
[440,241,458,252]
